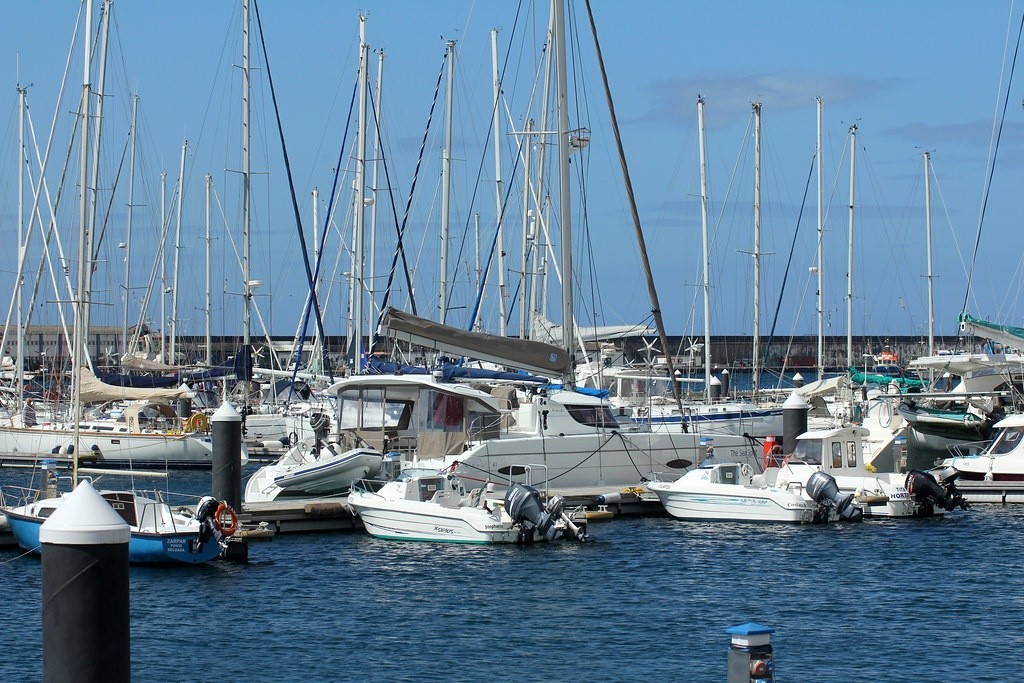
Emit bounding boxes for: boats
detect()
[648,456,864,524]
[0,482,243,565]
[772,424,971,520]
[349,460,589,543]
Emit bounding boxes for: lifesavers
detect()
[190,413,207,431]
[214,503,238,536]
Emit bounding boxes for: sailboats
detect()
[0,0,1024,492]
